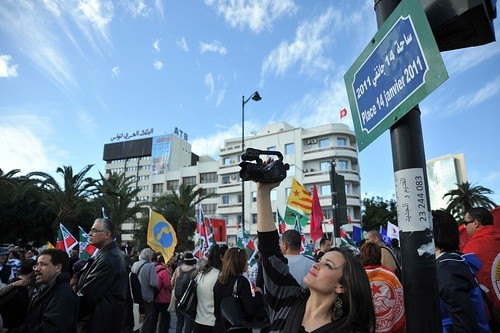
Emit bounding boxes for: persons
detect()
[0,207,500,333]
[256,157,377,333]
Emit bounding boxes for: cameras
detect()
[238,148,290,183]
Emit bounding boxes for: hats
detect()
[182,253,197,265]
[72,260,86,274]
[0,247,10,254]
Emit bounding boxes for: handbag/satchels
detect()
[177,279,198,322]
[129,262,148,303]
[393,268,403,285]
[487,298,499,333]
[221,276,267,333]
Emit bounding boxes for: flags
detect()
[56,176,400,262]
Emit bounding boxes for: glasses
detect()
[238,247,243,252]
[462,221,473,226]
[90,227,107,233]
[32,263,50,270]
[219,244,225,249]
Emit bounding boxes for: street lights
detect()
[241,91,262,231]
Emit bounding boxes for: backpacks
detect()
[175,266,196,300]
